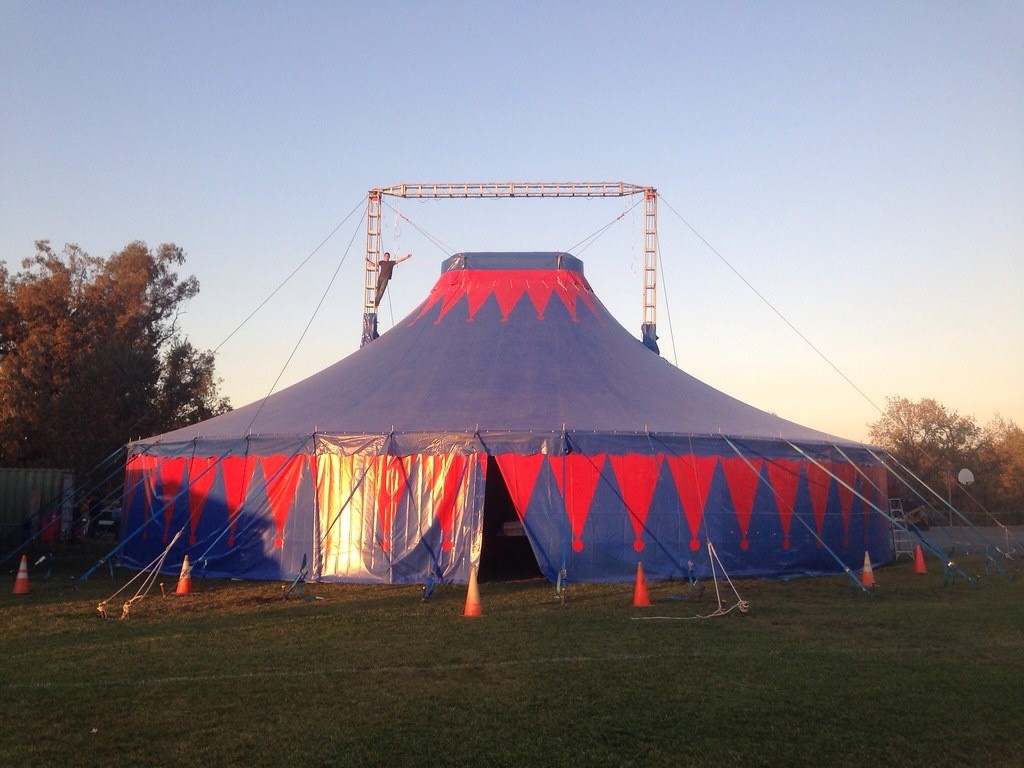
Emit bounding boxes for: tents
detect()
[118,251,895,585]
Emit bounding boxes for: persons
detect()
[98,498,120,534]
[365,253,413,308]
[80,495,93,536]
[87,494,101,537]
[112,505,121,541]
[37,513,61,565]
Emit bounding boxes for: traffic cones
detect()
[860,549,878,588]
[11,554,31,594]
[173,554,194,595]
[631,561,650,608]
[462,567,484,617]
[913,546,927,574]
